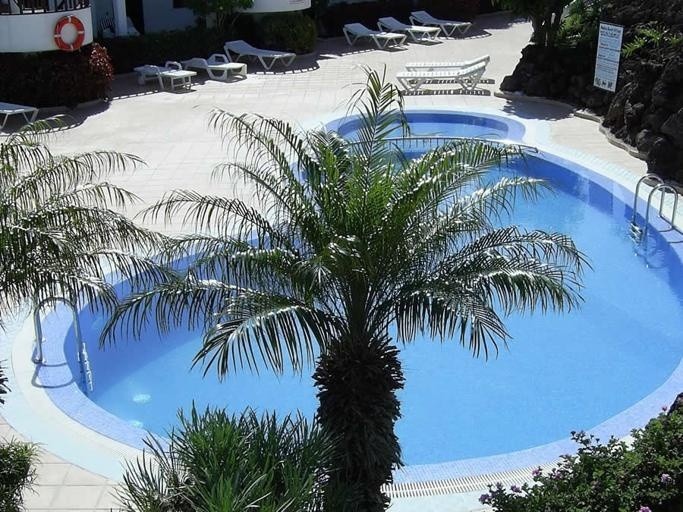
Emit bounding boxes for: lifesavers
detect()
[53,16,84,51]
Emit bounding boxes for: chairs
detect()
[377,16,442,43]
[342,23,407,50]
[133,60,197,94]
[224,40,297,70]
[409,11,472,37]
[0,101,40,129]
[182,53,247,83]
[396,54,492,95]
